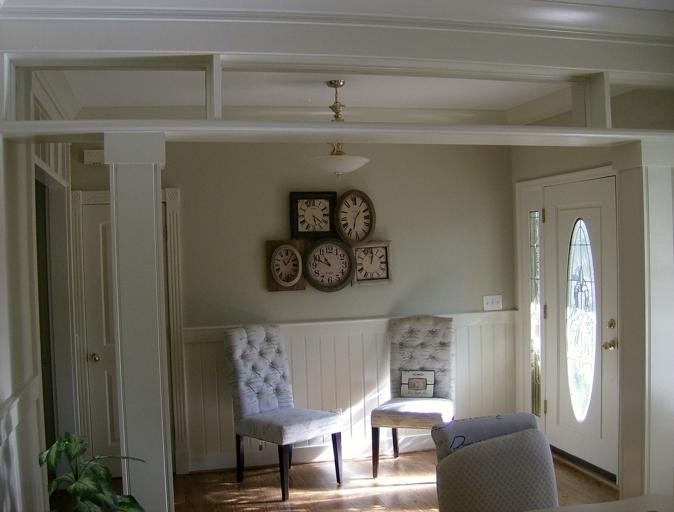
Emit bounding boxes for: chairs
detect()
[429,405,559,512]
[372,311,456,482]
[219,324,347,503]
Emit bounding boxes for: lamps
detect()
[309,78,370,177]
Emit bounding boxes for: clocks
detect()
[335,188,376,245]
[288,189,338,240]
[351,238,394,287]
[302,237,356,292]
[264,238,307,292]
